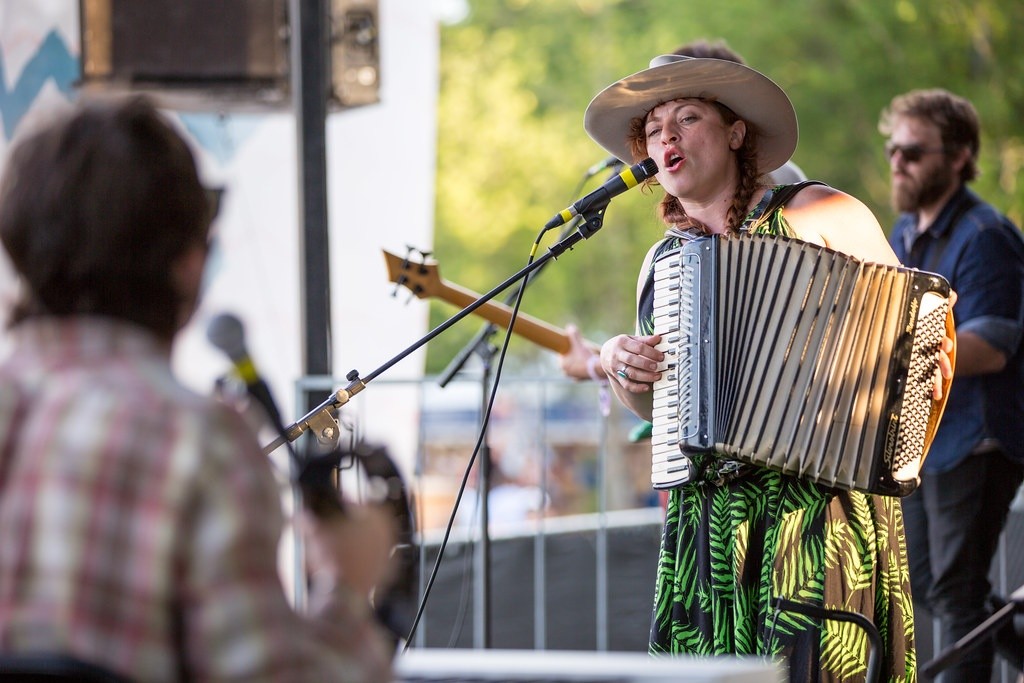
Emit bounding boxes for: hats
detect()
[584,54,799,178]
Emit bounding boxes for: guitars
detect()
[378,242,602,359]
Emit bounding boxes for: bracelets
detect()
[586,355,610,416]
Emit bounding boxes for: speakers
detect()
[79,0,382,114]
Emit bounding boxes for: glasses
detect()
[888,144,934,162]
[204,181,228,225]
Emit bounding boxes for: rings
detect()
[617,365,629,379]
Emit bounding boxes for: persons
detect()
[561,43,917,683]
[877,90,1024,683]
[0,96,403,683]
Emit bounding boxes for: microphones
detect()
[207,313,285,435]
[544,157,659,229]
[588,156,622,175]
